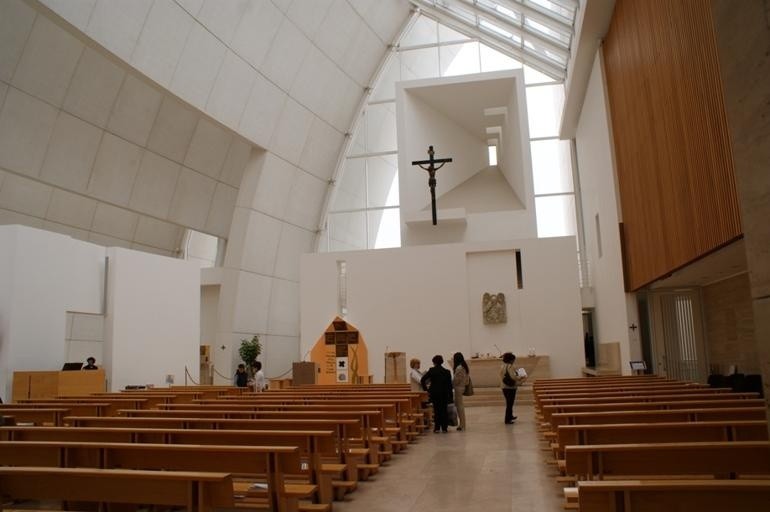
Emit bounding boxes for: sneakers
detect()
[442,428,448,432]
[433,429,440,434]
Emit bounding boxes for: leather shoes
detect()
[511,416,517,420]
[505,420,514,424]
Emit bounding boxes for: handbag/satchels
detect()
[447,405,459,426]
[463,376,474,397]
[502,366,519,387]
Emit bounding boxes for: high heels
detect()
[457,424,466,432]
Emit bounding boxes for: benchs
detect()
[0,377,433,512]
[532,374,769,512]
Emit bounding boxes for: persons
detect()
[498,352,528,424]
[418,161,447,190]
[83,357,98,370]
[451,352,471,431]
[246,361,265,393]
[233,363,248,387]
[409,358,432,431]
[420,355,454,433]
